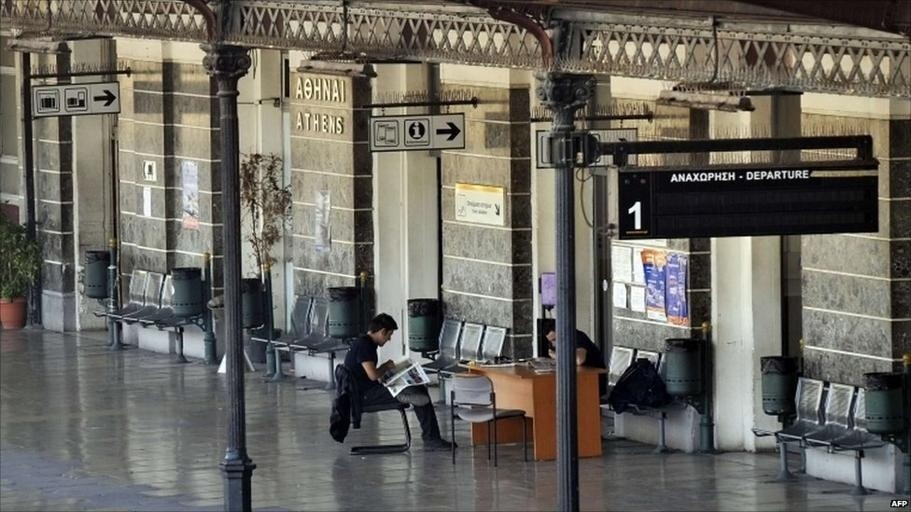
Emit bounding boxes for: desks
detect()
[458,359,608,461]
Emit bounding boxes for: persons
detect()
[343,312,458,451]
[545,327,607,397]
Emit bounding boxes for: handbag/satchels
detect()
[609,357,673,415]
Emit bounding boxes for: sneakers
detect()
[423,438,458,451]
[397,391,429,407]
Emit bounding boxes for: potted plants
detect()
[0,200,47,330]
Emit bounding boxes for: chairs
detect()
[309,311,351,389]
[454,326,508,376]
[123,272,164,322]
[653,352,677,454]
[445,372,527,465]
[599,346,635,408]
[290,297,329,354]
[268,295,312,383]
[752,377,823,482]
[141,274,190,364]
[441,322,485,375]
[334,364,411,455]
[422,318,462,407]
[636,351,661,366]
[105,268,149,350]
[833,387,890,495]
[806,384,854,444]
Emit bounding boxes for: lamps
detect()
[296,0,378,78]
[4,0,72,56]
[655,15,753,111]
